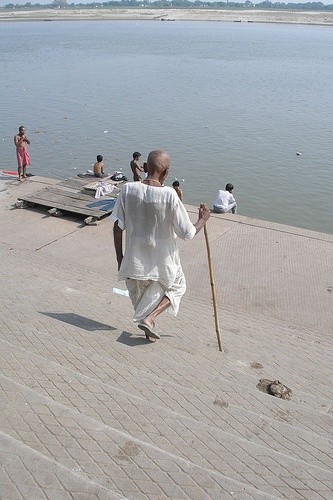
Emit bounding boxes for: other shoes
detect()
[138,318,160,339]
[146,332,158,343]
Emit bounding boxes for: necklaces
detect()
[143,179,160,184]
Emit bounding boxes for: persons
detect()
[110,151,211,341]
[94,155,110,177]
[130,152,148,181]
[14,126,31,181]
[172,181,183,202]
[213,183,237,214]
